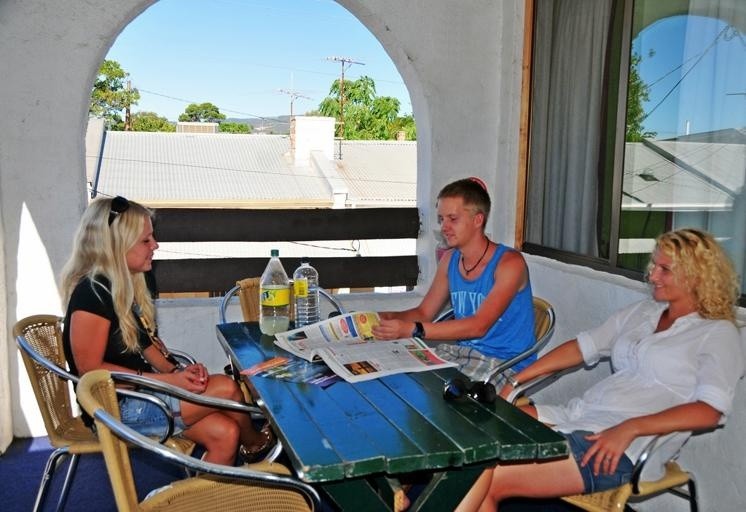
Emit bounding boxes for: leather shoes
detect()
[240,428,272,456]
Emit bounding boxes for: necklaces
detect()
[461,236,489,276]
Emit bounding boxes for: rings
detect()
[604,457,610,462]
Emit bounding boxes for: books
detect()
[272,311,459,385]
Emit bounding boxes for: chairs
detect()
[218,277,346,324]
[432,294,556,384]
[11,312,197,511]
[505,371,701,511]
[77,369,322,511]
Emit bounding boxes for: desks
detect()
[215,317,571,511]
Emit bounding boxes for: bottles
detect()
[256,247,292,337]
[292,256,321,330]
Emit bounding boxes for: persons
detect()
[373,178,539,512]
[454,225,745,512]
[56,196,284,478]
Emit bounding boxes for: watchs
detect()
[411,322,424,339]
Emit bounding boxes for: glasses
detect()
[443,377,498,405]
[107,195,129,226]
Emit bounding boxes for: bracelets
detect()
[508,376,519,388]
[168,366,179,373]
